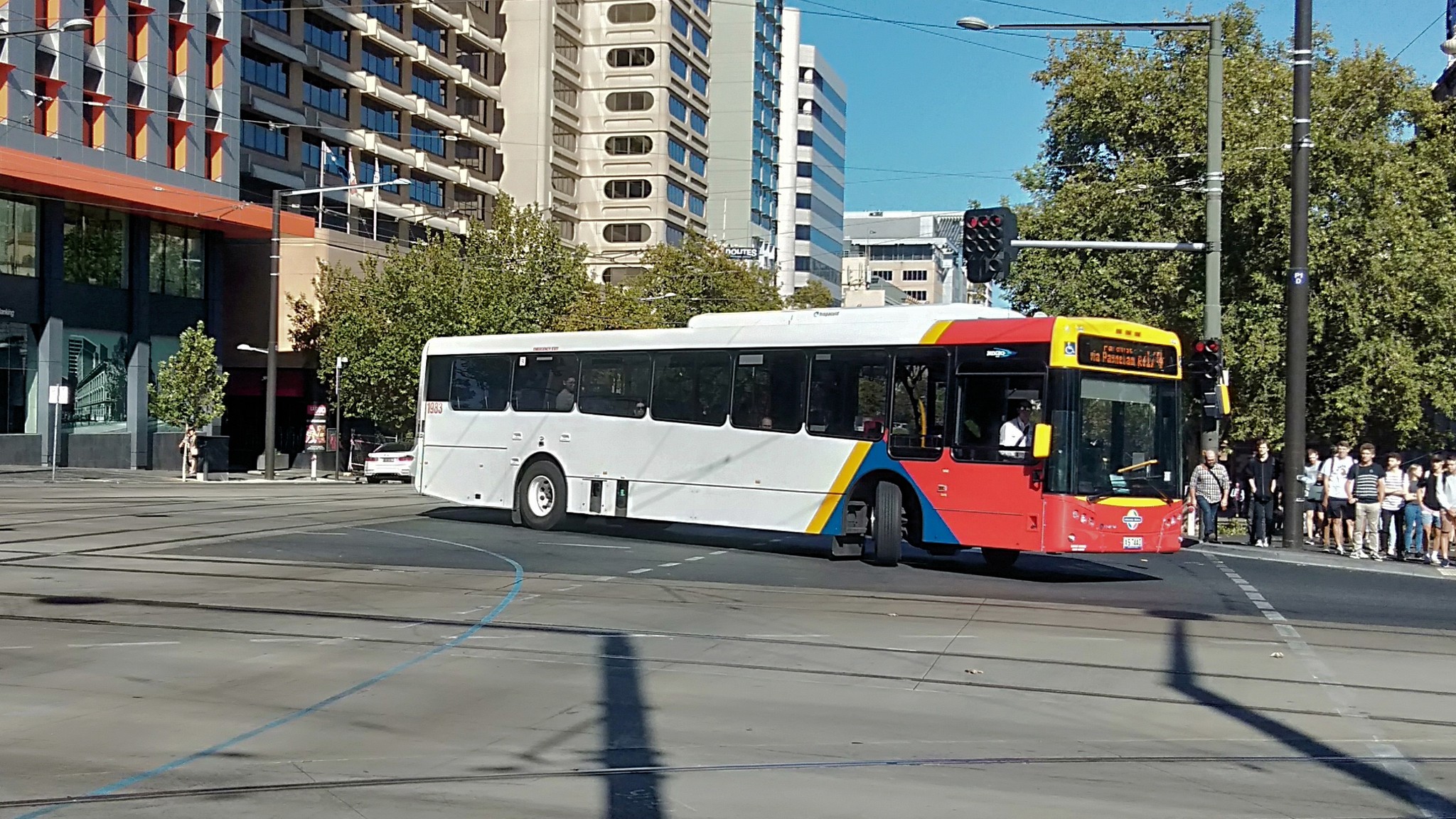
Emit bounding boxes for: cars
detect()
[363,439,418,483]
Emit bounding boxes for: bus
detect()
[411,290,1230,565]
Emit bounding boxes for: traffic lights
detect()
[1205,392,1222,419]
[963,206,1019,282]
[1193,336,1221,382]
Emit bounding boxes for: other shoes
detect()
[1371,553,1383,562]
[1204,533,1217,544]
[1322,544,1330,552]
[1405,550,1412,558]
[1336,547,1345,555]
[1350,550,1370,559]
[1305,536,1315,545]
[1423,551,1451,567]
[1414,552,1423,558]
[1397,552,1406,561]
[1257,537,1270,548]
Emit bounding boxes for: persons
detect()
[758,416,772,430]
[1189,450,1230,541]
[179,428,198,474]
[1230,481,1245,518]
[633,398,645,416]
[1245,438,1456,568]
[999,400,1035,460]
[554,375,575,410]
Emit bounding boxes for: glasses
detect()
[1020,405,1032,413]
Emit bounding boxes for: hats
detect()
[1432,453,1443,460]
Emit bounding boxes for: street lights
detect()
[238,177,412,479]
[957,16,1222,466]
